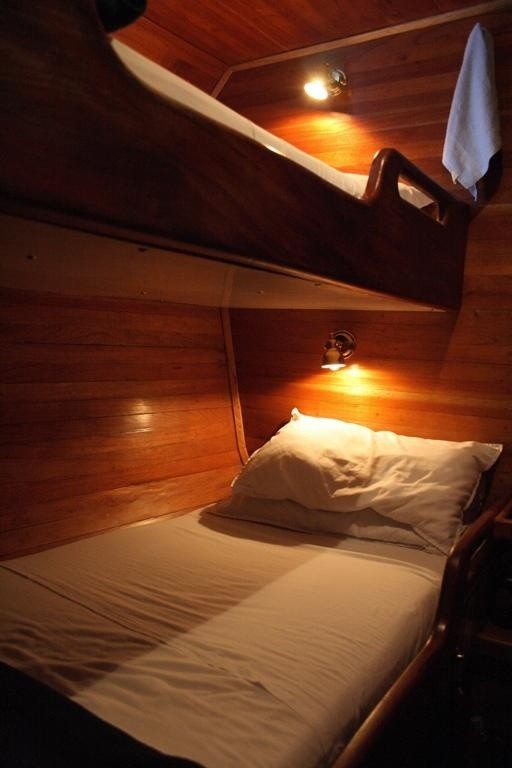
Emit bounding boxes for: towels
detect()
[443,21,501,200]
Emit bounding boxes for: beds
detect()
[1,493,512,767]
[2,0,471,312]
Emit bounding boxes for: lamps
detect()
[304,67,347,102]
[321,329,356,371]
